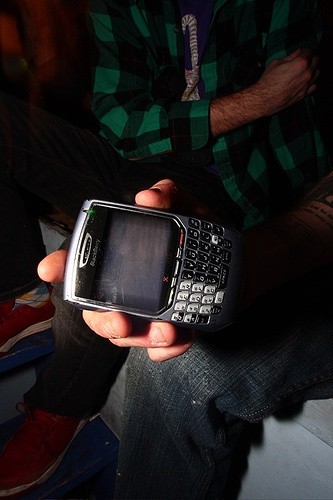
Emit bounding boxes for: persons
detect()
[37,170,333,500]
[0,0,331,500]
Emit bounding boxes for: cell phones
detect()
[62,198,247,332]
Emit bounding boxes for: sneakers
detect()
[0,284,55,358]
[0,401,89,497]
